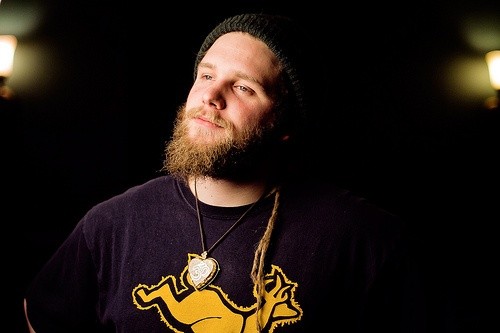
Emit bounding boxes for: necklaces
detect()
[188,175,267,291]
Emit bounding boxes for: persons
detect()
[21,12,452,333]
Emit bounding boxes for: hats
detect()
[194,13,306,110]
